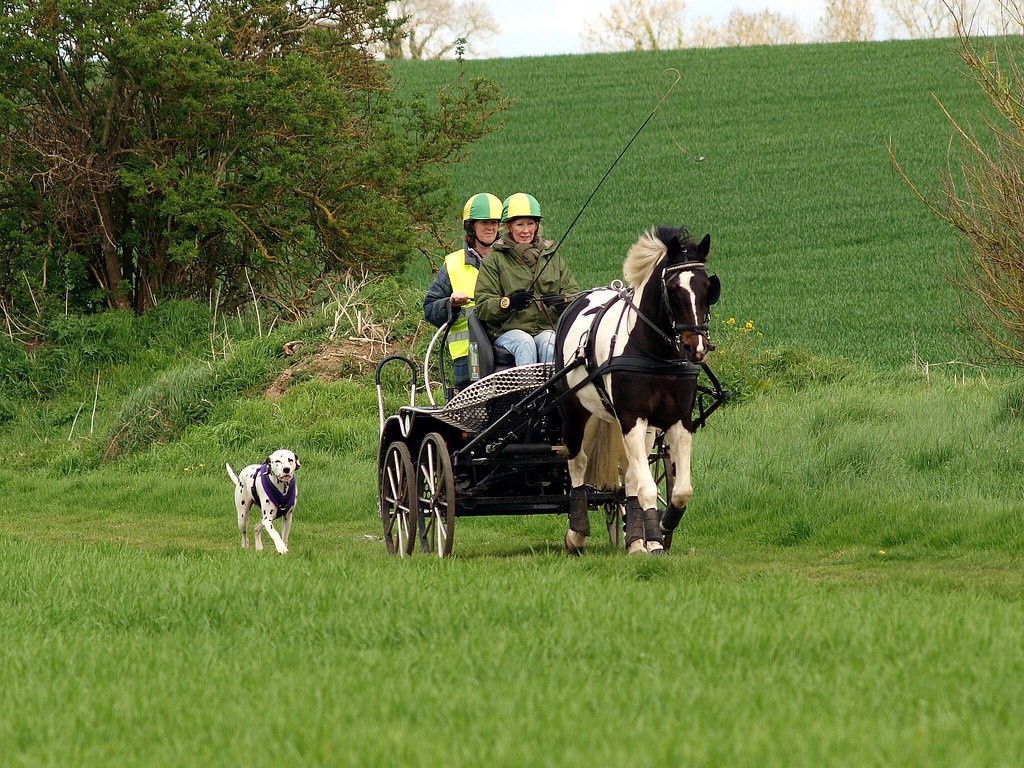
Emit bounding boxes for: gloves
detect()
[540,293,565,308]
[503,288,531,313]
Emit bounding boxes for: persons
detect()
[423,192,504,391]
[475,192,582,397]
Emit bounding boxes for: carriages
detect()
[375,222,722,557]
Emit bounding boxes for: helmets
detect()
[462,193,503,229]
[501,193,543,223]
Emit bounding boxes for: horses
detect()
[551,221,713,560]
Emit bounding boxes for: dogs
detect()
[224,448,303,556]
